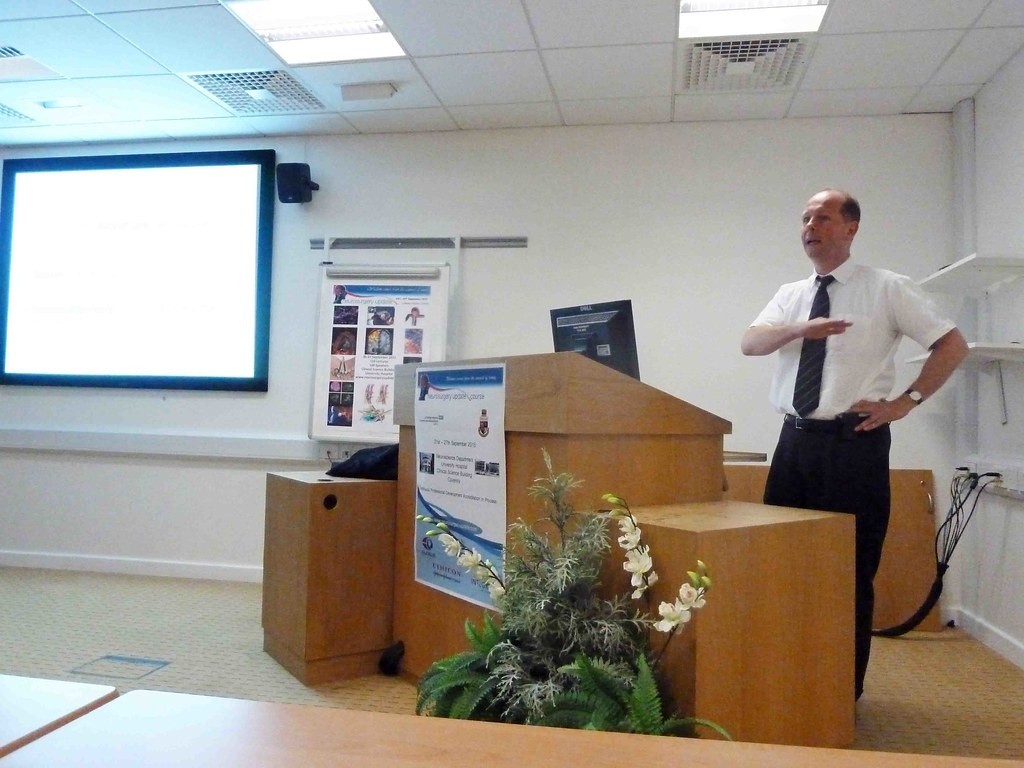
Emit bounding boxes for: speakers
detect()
[276,163,312,203]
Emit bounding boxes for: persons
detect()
[741,190,969,704]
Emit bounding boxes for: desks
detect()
[264,471,401,685]
[0,670,1023,767]
[591,500,853,750]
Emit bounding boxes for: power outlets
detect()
[954,459,1023,491]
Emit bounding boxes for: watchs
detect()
[904,389,923,404]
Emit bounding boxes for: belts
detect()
[784,414,860,432]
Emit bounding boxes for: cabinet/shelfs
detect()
[908,252,1024,366]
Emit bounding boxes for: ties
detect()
[792,276,835,418]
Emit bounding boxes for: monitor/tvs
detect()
[550,299,640,381]
